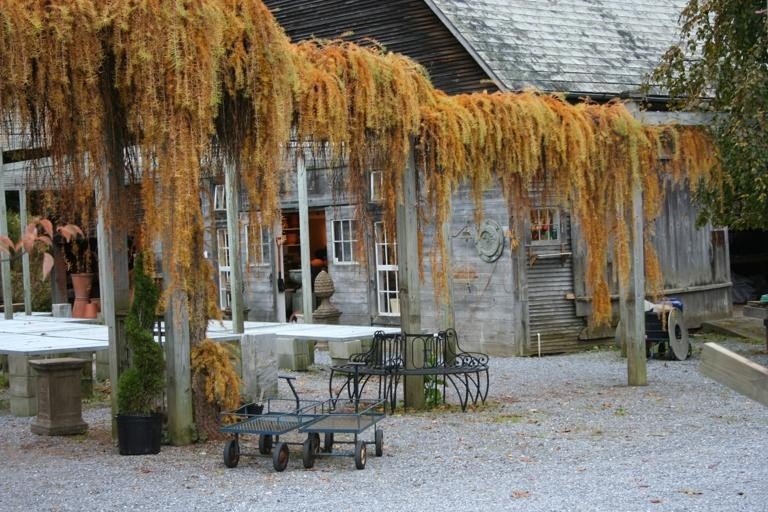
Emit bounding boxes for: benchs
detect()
[329,329,489,415]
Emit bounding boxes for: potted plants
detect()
[112,253,167,454]
